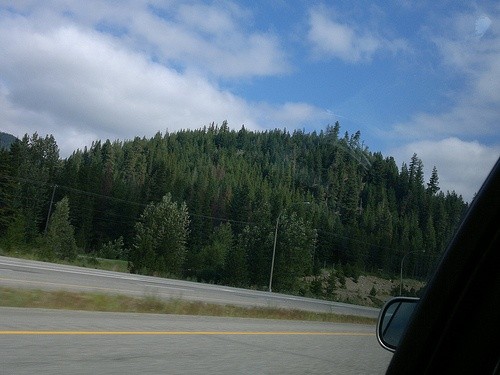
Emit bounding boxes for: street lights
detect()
[267,202,310,292]
[400,250,426,297]
